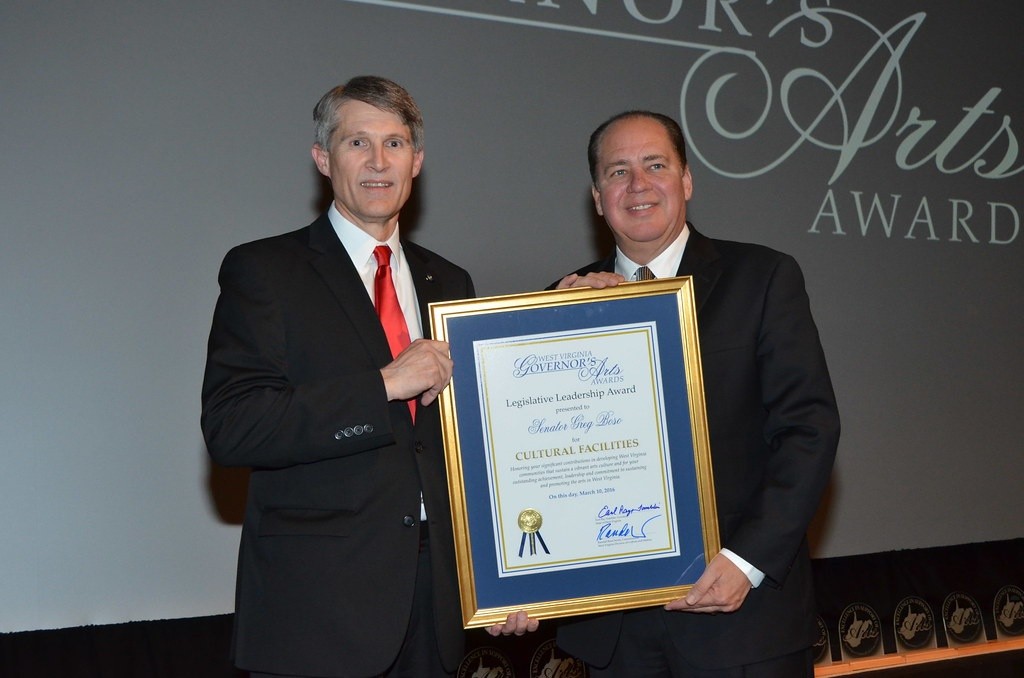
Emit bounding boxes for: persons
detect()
[201,75,538,678]
[544,109,841,678]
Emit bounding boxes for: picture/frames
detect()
[425,273,722,629]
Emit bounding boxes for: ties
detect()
[373,245,416,425]
[636,268,655,280]
